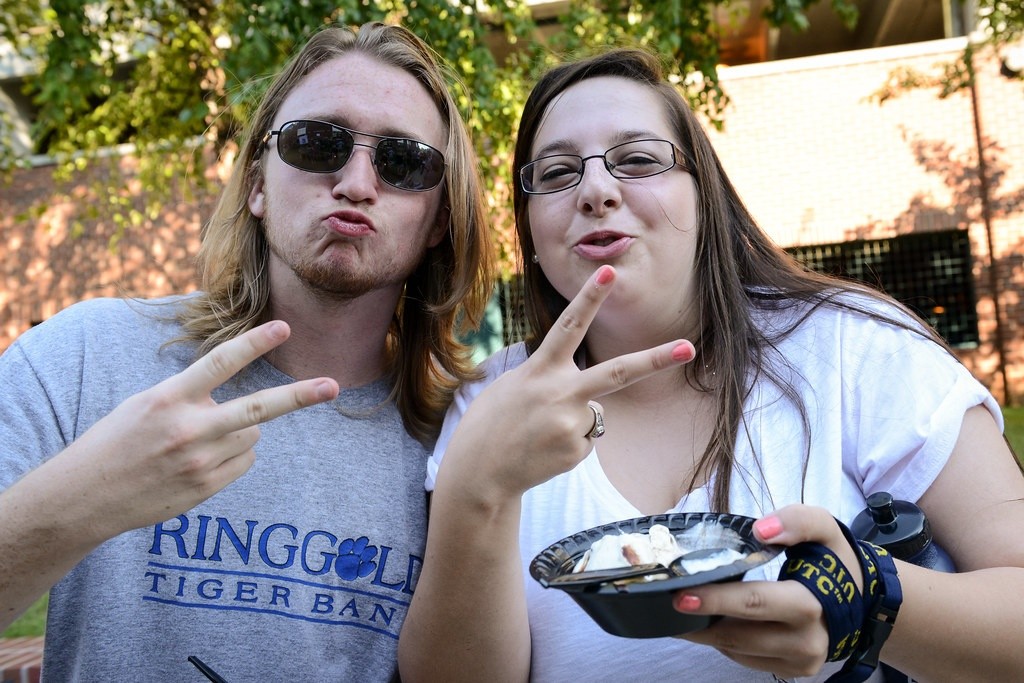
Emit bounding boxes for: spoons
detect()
[542,545,746,586]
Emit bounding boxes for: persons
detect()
[0,21,496,683]
[397,46,1024,683]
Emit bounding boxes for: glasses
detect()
[254,120,448,192]
[513,139,694,194]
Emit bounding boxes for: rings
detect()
[586,405,605,438]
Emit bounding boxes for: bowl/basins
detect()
[527,511,785,640]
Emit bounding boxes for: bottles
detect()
[848,489,961,683]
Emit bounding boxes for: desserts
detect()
[529,512,787,639]
